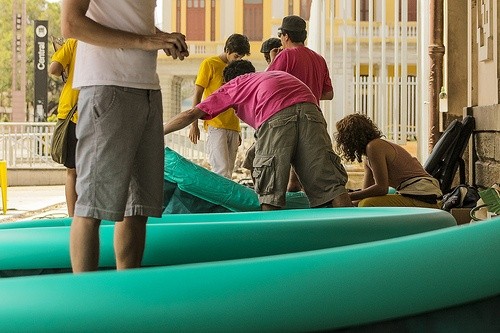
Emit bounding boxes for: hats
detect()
[278,15,306,32]
[260,37,283,52]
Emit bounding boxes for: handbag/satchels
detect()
[51,119,71,165]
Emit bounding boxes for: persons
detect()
[60,0,190,274]
[48,35,79,217]
[162,16,443,208]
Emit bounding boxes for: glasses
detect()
[278,31,284,37]
